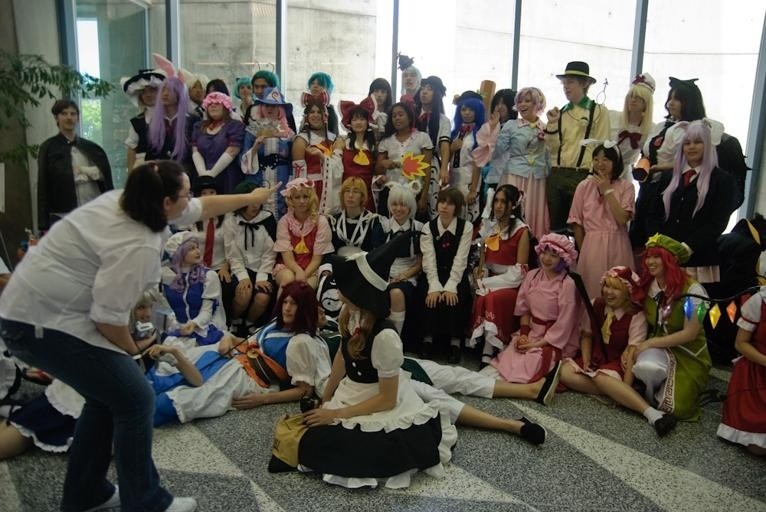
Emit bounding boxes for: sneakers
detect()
[446,345,463,364]
[465,346,478,361]
[166,496,200,511]
[479,354,492,370]
[517,416,547,445]
[654,410,675,438]
[537,360,562,405]
[62,484,120,510]
[421,342,435,353]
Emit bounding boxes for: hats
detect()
[555,61,596,83]
[603,266,640,295]
[164,230,199,259]
[632,73,656,93]
[203,91,232,110]
[331,231,426,315]
[645,232,688,262]
[254,87,293,113]
[119,69,167,109]
[280,177,316,198]
[535,232,578,266]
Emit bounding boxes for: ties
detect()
[204,217,215,268]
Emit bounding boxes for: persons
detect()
[664,114,732,371]
[1,53,656,490]
[0,159,286,512]
[708,211,766,367]
[556,262,677,438]
[626,75,748,277]
[620,231,723,424]
[2,159,286,512]
[717,277,766,456]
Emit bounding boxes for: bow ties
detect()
[617,130,642,150]
[239,221,259,250]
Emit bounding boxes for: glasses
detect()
[176,193,193,202]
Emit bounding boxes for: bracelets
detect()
[604,188,614,196]
